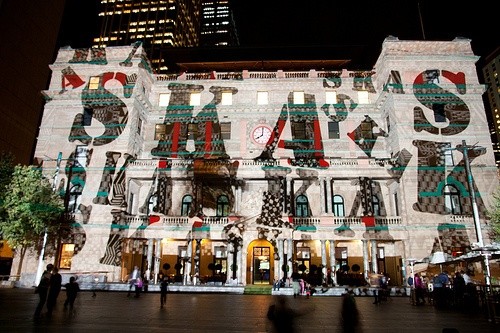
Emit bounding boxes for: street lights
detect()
[438,140,493,298]
[33,152,78,287]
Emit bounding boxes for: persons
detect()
[414,270,479,310]
[160,280,168,308]
[44,267,62,318]
[32,264,54,322]
[63,277,79,318]
[134,276,143,297]
[143,277,149,294]
[128,266,140,295]
[274,280,280,291]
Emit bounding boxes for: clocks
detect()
[250,124,273,147]
